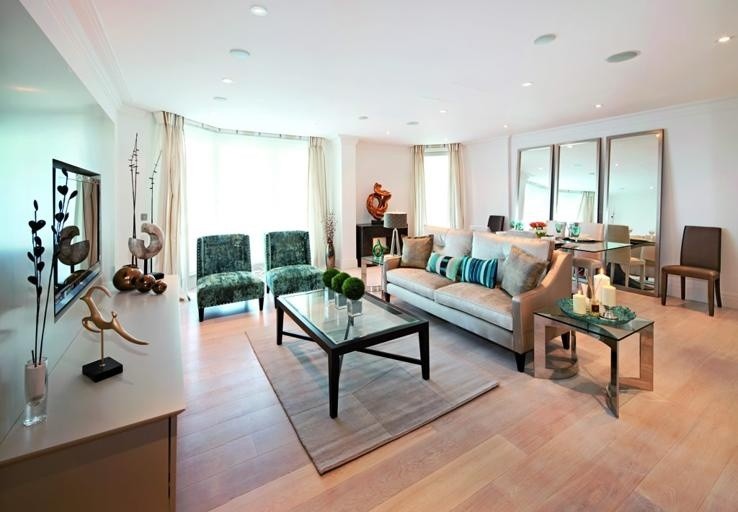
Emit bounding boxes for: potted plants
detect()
[331,272,352,310]
[341,277,366,317]
[321,268,341,304]
[21,165,93,429]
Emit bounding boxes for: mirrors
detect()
[554,137,600,224]
[603,129,664,296]
[516,143,553,223]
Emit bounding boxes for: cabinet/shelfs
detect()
[1,273,189,512]
[356,224,408,269]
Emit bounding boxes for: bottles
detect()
[372,238,383,261]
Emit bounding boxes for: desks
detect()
[360,254,402,303]
[546,237,633,257]
[533,298,656,419]
[630,237,657,250]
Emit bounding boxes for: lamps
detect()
[383,212,407,256]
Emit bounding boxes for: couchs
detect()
[380,224,573,373]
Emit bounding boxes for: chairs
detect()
[605,225,647,291]
[484,215,505,233]
[663,226,725,317]
[264,230,324,310]
[195,234,267,323]
[496,224,608,299]
[641,232,657,282]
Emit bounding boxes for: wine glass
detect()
[509,219,581,245]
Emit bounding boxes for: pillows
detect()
[399,233,433,268]
[500,244,550,298]
[457,255,498,291]
[425,251,464,283]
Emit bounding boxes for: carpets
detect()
[245,313,499,477]
